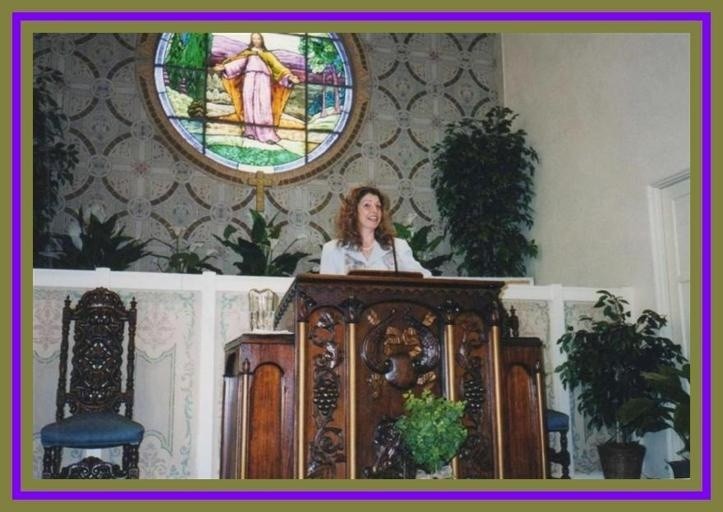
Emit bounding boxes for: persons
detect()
[319,187,432,279]
[212,32,301,145]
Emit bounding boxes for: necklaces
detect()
[362,243,374,250]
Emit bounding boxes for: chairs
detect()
[501,298,570,479]
[41,287,145,479]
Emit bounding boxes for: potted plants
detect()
[614,363,690,479]
[553,290,688,479]
